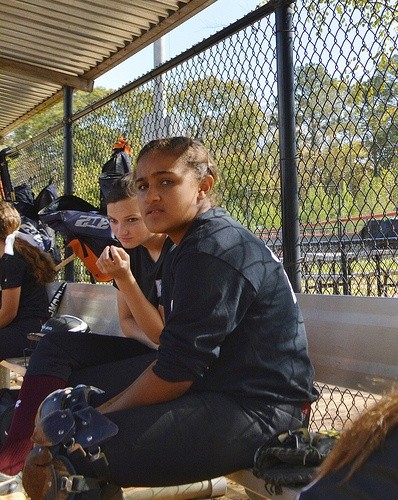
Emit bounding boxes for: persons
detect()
[0,172,173,475]
[22,136,321,500]
[2,198,60,362]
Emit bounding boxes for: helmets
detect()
[27,314,92,342]
[34,384,115,427]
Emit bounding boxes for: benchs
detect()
[0,284,398,500]
[301,270,398,281]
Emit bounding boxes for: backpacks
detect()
[9,182,63,276]
[38,149,133,284]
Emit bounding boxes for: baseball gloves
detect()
[251,426,343,485]
[32,385,121,448]
[21,445,117,500]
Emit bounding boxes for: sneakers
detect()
[0,470,32,500]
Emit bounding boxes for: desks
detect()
[300,249,398,295]
